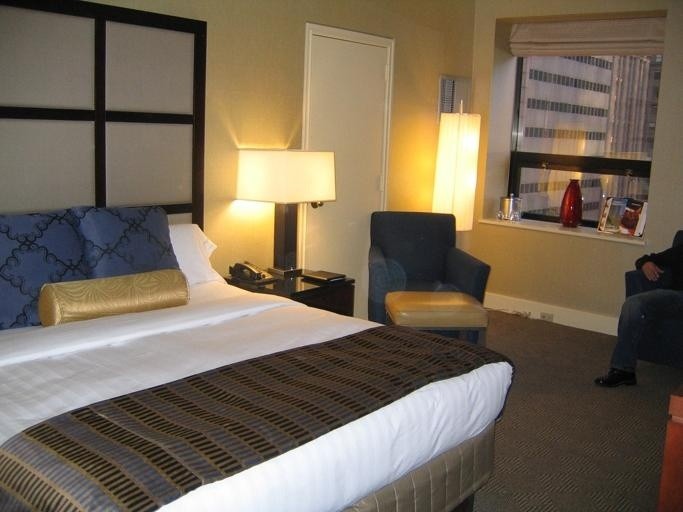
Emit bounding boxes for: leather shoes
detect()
[594,368,636,388]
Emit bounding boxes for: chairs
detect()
[367,210,490,344]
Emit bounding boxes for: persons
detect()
[594,228,681,388]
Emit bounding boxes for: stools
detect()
[383,290,488,345]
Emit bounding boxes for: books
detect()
[595,195,648,237]
[302,270,347,285]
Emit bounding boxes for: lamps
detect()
[235,149,337,279]
[432,98,482,233]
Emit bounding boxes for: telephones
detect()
[228,261,278,283]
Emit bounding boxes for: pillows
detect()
[0,204,190,331]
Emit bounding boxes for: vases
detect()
[559,178,583,229]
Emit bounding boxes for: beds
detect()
[0,223,516,512]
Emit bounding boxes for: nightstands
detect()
[222,268,355,319]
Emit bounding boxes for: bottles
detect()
[498,193,523,221]
